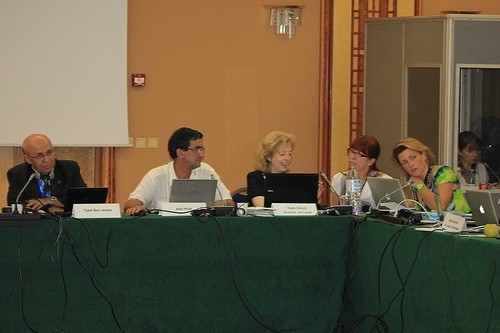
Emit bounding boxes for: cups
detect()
[11,204,23,214]
[236,203,248,216]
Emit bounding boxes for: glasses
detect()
[25,150,53,159]
[187,147,205,153]
[347,149,372,159]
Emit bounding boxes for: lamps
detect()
[270,7,301,39]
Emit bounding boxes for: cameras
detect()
[148,209,159,214]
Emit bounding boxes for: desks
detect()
[1,211,500,333]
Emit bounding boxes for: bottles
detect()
[346,165,360,215]
[465,168,479,191]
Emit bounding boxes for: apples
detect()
[484,223,499,238]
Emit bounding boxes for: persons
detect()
[7,134,87,214]
[390,137,475,226]
[330,135,392,205]
[246,131,325,208]
[123,127,236,216]
[456,131,489,186]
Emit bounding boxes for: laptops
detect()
[366,176,436,223]
[465,189,500,226]
[263,172,319,208]
[169,179,217,207]
[56,187,108,216]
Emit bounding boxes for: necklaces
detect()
[424,165,432,185]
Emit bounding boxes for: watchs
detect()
[416,184,425,191]
[50,196,57,205]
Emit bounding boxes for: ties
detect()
[40,175,52,212]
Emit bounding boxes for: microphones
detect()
[393,200,443,223]
[211,174,224,206]
[376,182,412,209]
[480,158,500,185]
[320,171,340,205]
[15,173,34,203]
